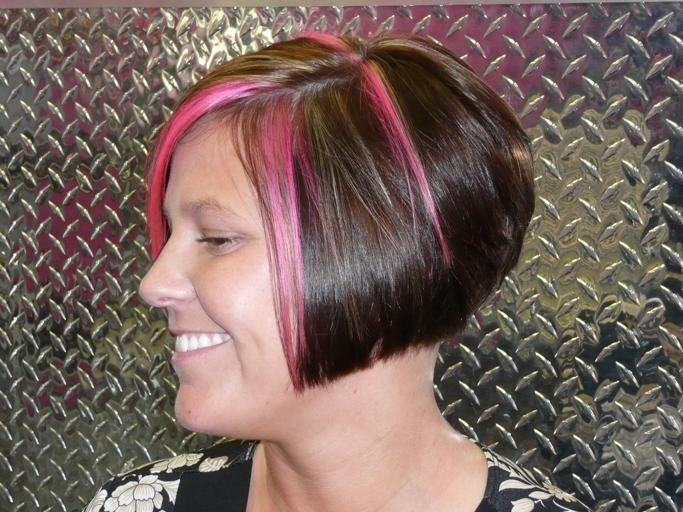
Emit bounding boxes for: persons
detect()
[80,32,594,511]
